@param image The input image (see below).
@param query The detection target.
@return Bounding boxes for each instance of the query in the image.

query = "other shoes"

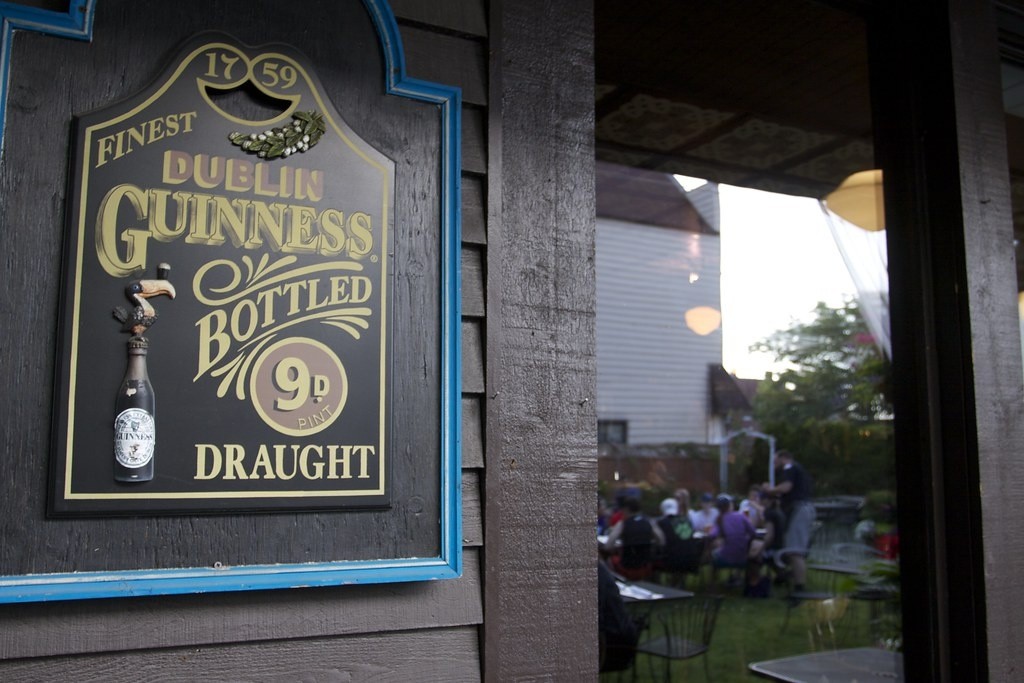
[726,573,737,587]
[788,594,805,610]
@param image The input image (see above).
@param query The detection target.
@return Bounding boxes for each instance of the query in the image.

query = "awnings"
[713,364,751,414]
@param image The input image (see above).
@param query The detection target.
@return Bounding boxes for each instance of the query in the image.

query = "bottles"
[113,336,155,482]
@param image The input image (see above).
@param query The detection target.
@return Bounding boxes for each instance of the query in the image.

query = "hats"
[716,493,734,505]
[701,493,713,502]
[660,498,678,516]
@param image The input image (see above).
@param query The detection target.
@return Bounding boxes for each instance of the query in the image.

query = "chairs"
[611,496,893,683]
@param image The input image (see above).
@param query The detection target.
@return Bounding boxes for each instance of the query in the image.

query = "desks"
[613,579,695,683]
[805,560,895,647]
[814,502,853,548]
[746,647,906,683]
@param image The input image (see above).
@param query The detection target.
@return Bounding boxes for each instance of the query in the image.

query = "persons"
[597,451,816,607]
